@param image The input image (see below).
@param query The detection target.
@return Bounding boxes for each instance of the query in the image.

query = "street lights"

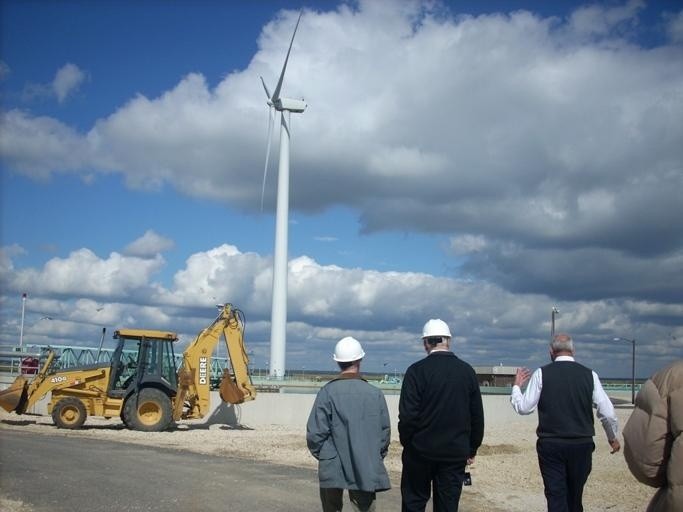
[550,306,560,344]
[18,293,27,353]
[613,337,635,404]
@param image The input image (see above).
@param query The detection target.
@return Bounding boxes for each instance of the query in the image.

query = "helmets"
[333,336,365,363]
[419,319,452,339]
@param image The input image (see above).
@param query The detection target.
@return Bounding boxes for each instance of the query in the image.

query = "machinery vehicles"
[0,304,256,433]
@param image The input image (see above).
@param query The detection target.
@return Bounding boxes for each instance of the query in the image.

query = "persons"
[306,337,391,512]
[398,318,484,512]
[622,360,683,512]
[509,334,620,512]
